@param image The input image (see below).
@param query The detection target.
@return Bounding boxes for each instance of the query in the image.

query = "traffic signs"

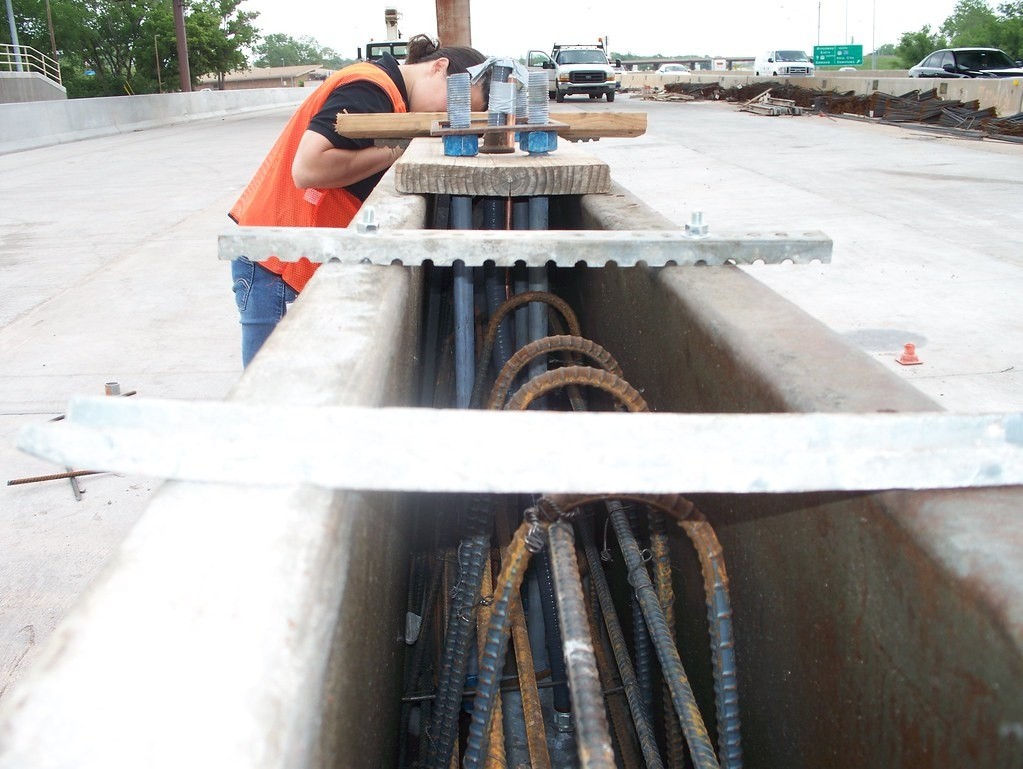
[814,45,864,67]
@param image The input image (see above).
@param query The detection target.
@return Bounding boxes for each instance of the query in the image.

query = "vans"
[753,49,815,78]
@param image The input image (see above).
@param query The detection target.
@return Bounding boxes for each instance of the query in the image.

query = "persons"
[230,32,493,370]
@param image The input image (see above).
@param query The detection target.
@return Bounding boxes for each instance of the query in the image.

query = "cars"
[655,63,692,75]
[908,47,1023,78]
[838,68,857,71]
[609,64,625,72]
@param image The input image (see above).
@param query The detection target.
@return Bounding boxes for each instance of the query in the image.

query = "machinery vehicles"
[354,41,413,65]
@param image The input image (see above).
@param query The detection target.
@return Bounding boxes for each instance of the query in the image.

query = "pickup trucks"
[526,36,616,102]
